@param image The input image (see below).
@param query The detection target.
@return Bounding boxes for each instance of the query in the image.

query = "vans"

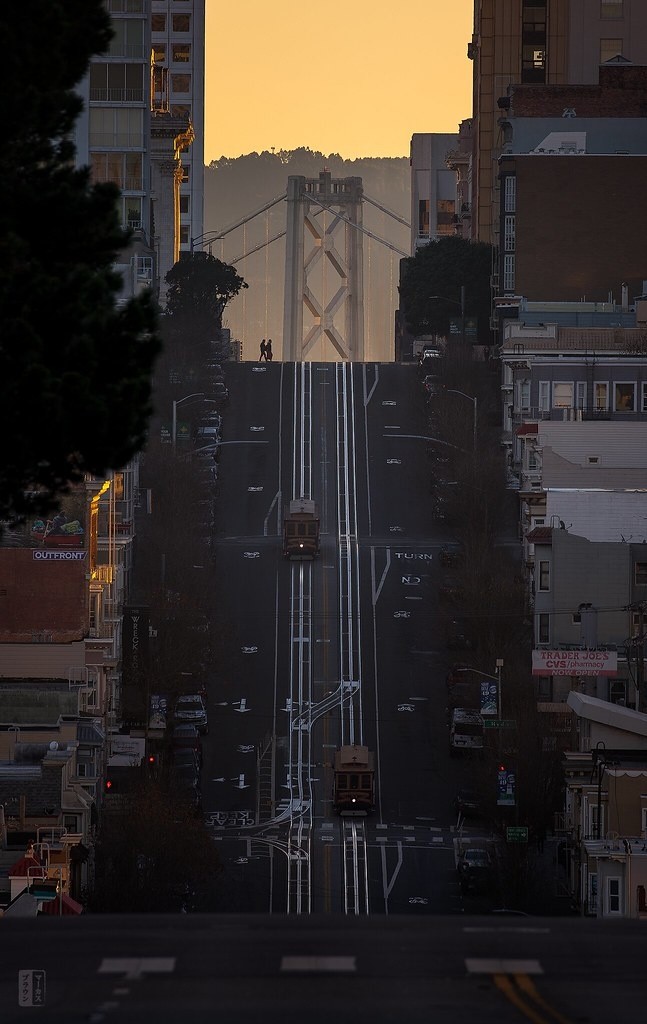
[450,708,485,758]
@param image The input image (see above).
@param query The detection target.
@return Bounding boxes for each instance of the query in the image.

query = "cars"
[456,848,493,881]
[454,790,485,818]
[159,353,230,915]
[417,345,481,709]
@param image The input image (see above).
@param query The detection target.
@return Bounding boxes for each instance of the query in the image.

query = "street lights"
[172,391,270,462]
[447,481,488,535]
[381,388,478,523]
[456,669,502,762]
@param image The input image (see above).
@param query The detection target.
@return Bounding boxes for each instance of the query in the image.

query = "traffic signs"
[507,826,528,843]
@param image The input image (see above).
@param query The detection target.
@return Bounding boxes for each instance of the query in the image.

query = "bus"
[283,499,322,561]
[332,744,376,817]
